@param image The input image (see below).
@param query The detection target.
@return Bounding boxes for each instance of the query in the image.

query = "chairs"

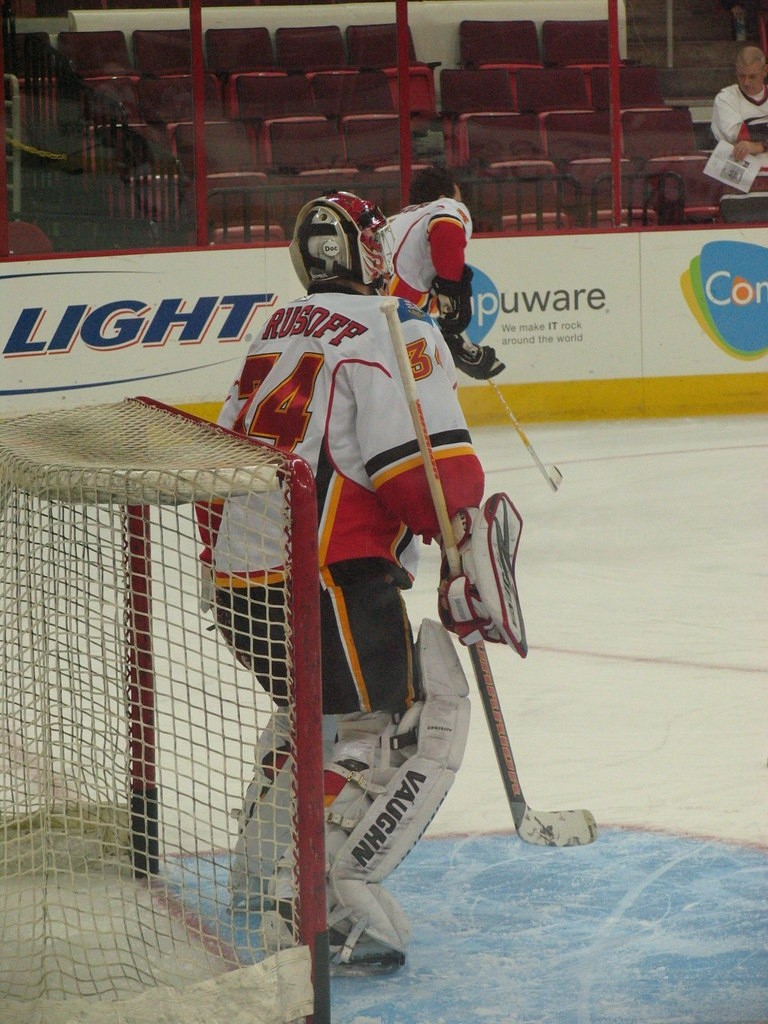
[0,21,767,256]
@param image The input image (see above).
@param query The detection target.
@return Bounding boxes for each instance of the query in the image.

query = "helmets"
[288,191,397,294]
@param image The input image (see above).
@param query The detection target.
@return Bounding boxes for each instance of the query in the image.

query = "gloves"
[432,263,473,335]
[443,332,506,381]
[436,506,506,646]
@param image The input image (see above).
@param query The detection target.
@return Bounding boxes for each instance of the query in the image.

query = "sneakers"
[260,880,405,977]
[226,874,268,916]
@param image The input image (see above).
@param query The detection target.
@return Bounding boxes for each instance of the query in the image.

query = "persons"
[385,170,506,379]
[711,47,768,222]
[721,0,768,43]
[197,193,528,975]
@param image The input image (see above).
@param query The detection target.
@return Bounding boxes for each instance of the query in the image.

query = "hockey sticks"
[379,298,597,847]
[488,378,561,490]
